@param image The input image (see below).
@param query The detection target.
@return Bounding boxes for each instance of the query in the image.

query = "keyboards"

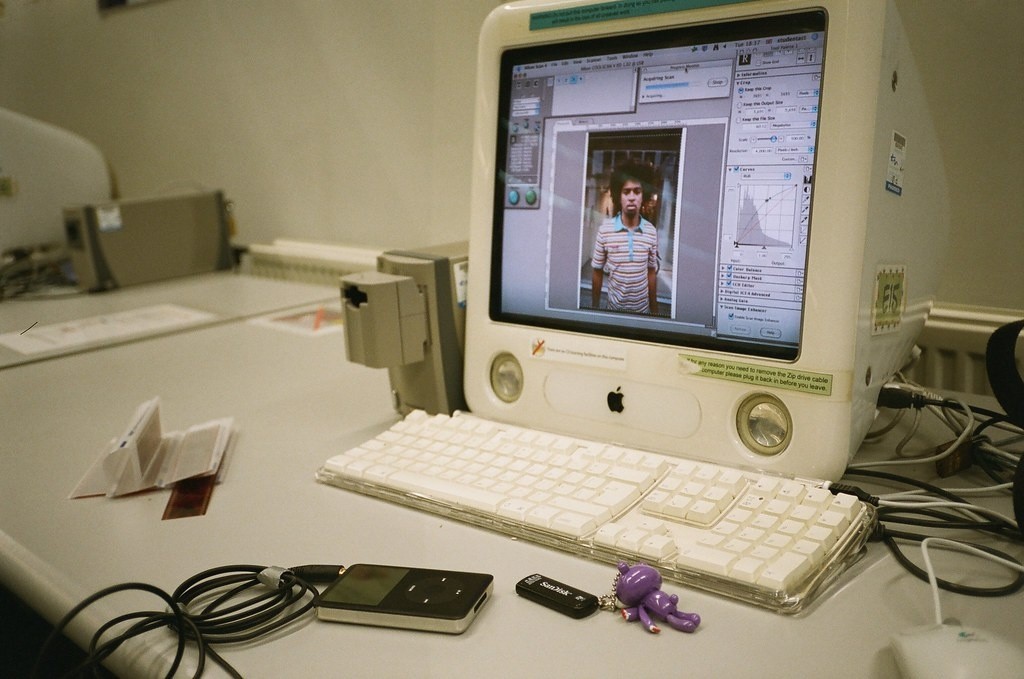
[317,405,873,614]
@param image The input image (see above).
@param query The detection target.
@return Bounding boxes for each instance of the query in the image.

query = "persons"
[591,169,659,315]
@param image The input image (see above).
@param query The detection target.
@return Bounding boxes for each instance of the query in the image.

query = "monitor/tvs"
[0,109,115,281]
[463,0,948,487]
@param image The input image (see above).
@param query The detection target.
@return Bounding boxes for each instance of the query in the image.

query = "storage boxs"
[61,190,237,293]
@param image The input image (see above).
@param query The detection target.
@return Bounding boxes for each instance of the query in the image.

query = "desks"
[0,271,1024,679]
